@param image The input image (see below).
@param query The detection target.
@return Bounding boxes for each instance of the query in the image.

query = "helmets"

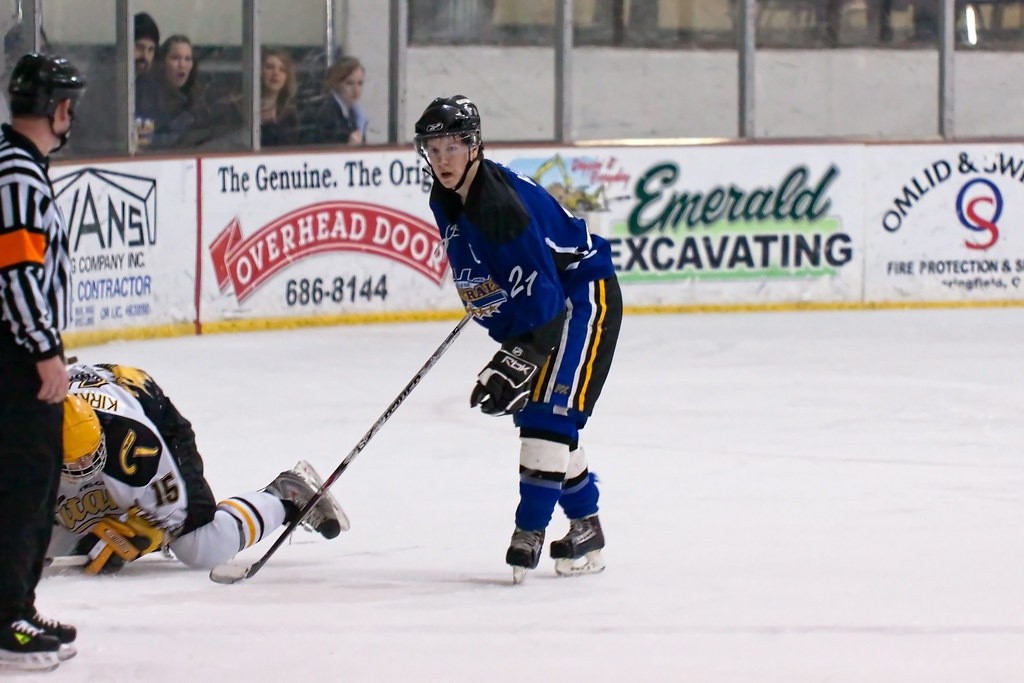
[9,51,86,117]
[413,95,480,159]
[61,394,107,483]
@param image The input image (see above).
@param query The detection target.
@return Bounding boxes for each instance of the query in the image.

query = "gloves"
[75,510,160,576]
[471,333,547,415]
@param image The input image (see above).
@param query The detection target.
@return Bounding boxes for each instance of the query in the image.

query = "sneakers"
[30,613,77,661]
[259,458,349,540]
[549,516,605,576]
[505,525,545,584]
[1,620,61,671]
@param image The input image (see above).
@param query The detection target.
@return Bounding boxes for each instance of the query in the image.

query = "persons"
[0,21,72,158]
[308,57,369,146]
[225,45,302,150]
[70,12,162,158]
[42,364,350,573]
[0,54,88,673]
[413,95,623,586]
[147,33,225,150]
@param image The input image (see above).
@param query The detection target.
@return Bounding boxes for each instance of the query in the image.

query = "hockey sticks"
[43,554,92,568]
[208,308,476,587]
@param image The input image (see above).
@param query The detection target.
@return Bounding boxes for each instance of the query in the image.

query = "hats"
[134,12,161,47]
